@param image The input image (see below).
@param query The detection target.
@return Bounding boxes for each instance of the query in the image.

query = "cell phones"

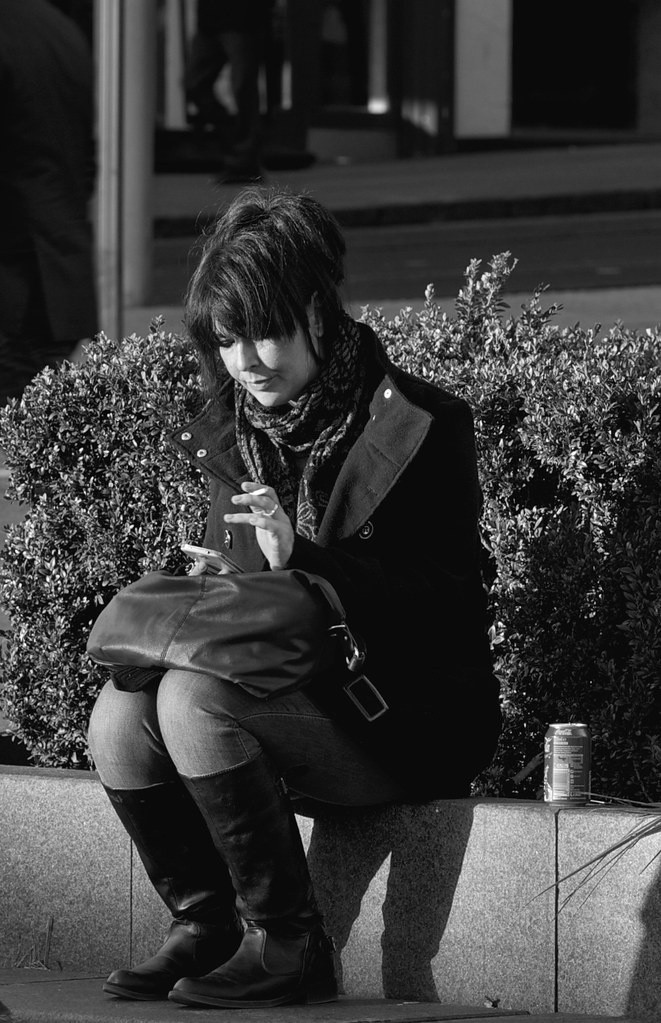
[180,545,243,575]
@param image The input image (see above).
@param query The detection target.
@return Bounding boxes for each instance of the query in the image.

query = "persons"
[89,189,501,1007]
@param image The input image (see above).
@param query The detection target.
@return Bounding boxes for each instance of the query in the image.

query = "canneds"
[543,721,592,808]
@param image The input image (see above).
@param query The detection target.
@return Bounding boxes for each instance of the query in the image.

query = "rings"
[262,503,279,518]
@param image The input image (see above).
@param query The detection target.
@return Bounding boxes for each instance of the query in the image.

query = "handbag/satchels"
[87,572,361,698]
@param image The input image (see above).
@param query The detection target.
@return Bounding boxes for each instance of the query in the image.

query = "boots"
[168,747,336,1007]
[103,778,246,999]
